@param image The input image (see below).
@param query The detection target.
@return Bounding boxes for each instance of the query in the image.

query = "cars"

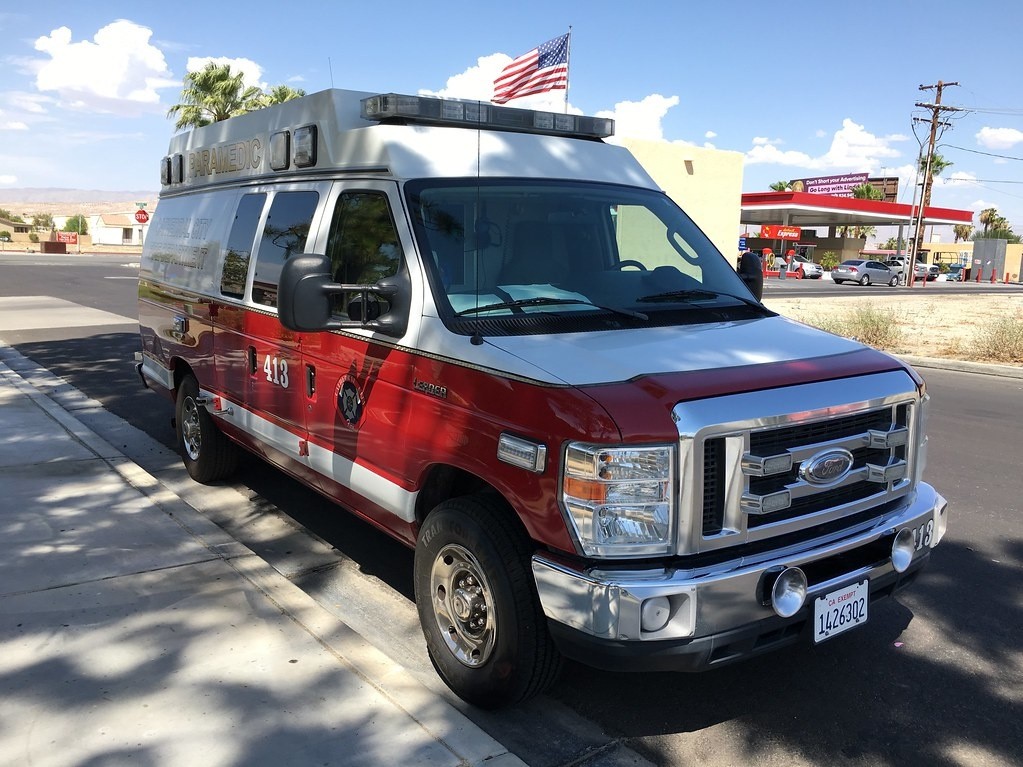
[882,260,919,281]
[831,259,900,287]
[773,254,824,279]
[889,256,940,281]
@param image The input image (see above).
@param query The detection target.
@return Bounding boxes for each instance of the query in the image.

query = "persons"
[354,215,400,273]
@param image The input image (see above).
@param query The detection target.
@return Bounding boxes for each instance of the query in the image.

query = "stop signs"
[135,210,149,223]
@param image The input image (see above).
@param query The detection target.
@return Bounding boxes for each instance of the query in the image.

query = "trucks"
[946,264,967,281]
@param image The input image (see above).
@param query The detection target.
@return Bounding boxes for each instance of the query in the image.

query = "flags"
[490,33,569,104]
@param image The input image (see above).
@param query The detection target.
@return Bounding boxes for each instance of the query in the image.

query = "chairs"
[503,221,603,295]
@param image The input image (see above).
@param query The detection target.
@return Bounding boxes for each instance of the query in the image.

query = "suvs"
[135,87,949,711]
